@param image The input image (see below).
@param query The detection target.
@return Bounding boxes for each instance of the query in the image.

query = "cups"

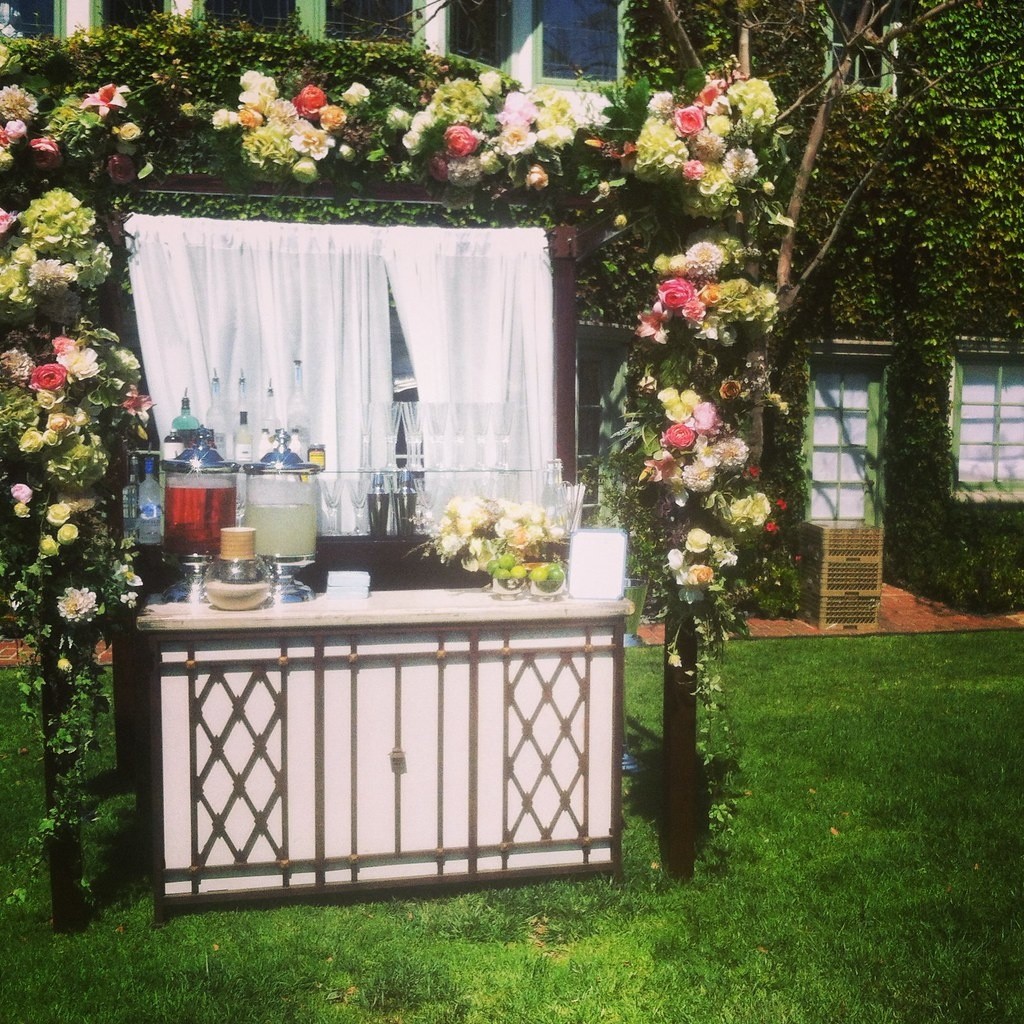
[355,401,513,470]
[320,476,585,537]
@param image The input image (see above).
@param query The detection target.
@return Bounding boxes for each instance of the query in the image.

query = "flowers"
[1,24,800,905]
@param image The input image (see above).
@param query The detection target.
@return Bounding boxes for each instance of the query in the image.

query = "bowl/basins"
[530,580,566,601]
[492,579,525,600]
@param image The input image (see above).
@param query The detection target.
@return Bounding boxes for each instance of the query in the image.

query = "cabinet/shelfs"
[113,591,637,928]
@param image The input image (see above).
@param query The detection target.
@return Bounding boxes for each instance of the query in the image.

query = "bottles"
[233,378,254,431]
[260,388,279,435]
[393,472,417,535]
[287,360,310,443]
[122,456,141,537]
[367,474,390,536]
[205,376,226,456]
[139,458,161,544]
[259,429,273,459]
[204,526,271,610]
[172,397,200,447]
[164,429,185,460]
[542,458,565,531]
[233,412,253,461]
[287,428,302,457]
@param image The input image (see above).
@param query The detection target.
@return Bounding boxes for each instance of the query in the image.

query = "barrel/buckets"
[623,578,648,635]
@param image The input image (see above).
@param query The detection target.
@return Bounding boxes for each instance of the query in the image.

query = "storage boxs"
[799,555,882,598]
[800,521,885,564]
[799,586,881,632]
